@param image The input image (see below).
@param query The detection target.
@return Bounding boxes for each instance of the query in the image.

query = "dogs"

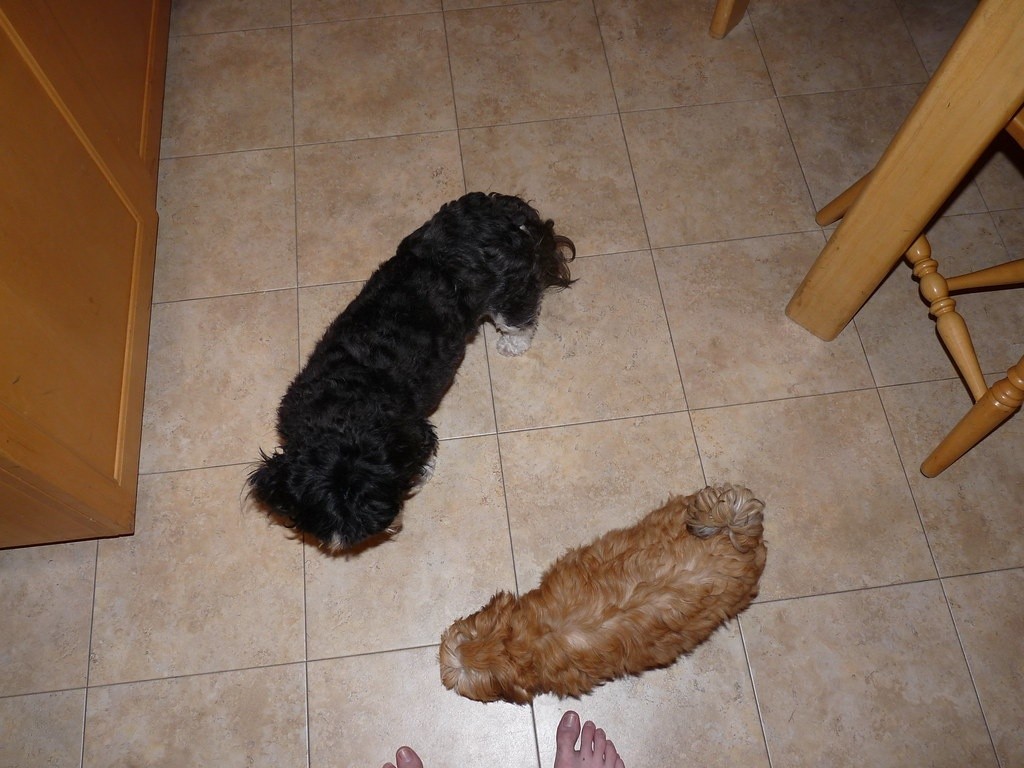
[248,188,578,559]
[439,484,768,706]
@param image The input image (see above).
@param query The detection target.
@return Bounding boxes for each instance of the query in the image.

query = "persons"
[383,710,625,768]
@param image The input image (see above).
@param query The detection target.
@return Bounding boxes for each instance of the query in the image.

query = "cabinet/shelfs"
[0,0,174,551]
